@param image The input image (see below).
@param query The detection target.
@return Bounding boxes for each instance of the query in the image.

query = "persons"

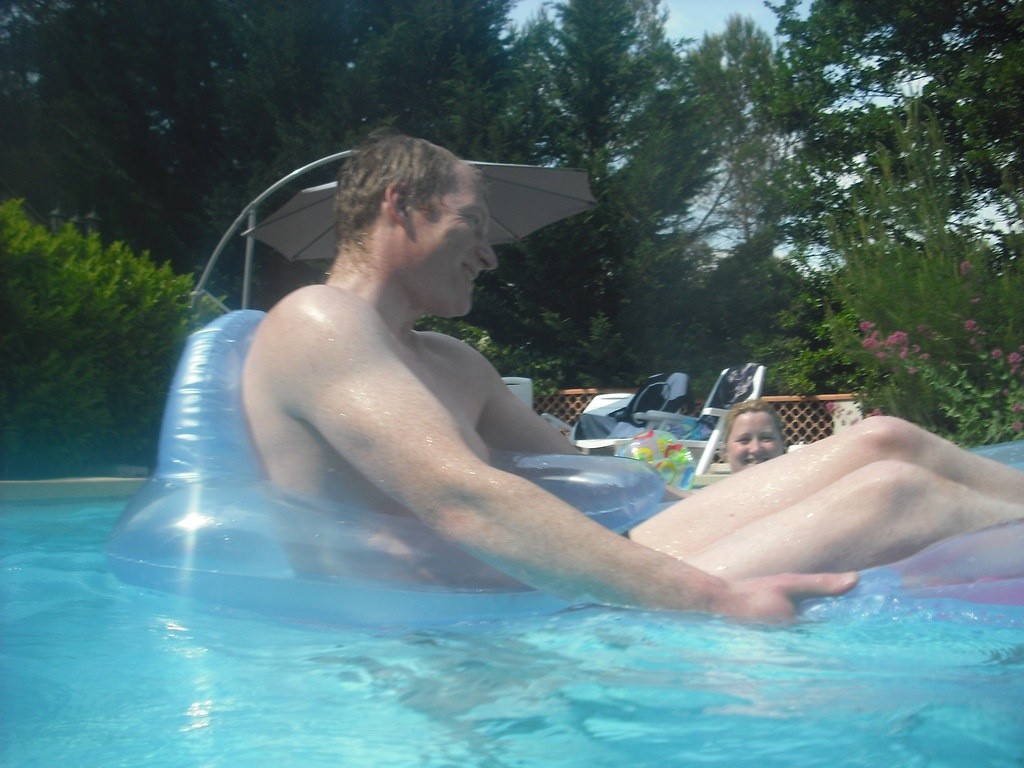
[243,124,1023,631]
[724,401,786,474]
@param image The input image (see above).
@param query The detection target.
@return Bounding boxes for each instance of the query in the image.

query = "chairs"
[569,371,689,457]
[540,393,635,440]
[646,362,771,476]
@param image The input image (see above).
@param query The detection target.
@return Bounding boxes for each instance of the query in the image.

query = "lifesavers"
[107,306,1022,605]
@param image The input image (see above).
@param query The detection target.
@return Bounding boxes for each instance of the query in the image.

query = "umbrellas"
[241,160,599,262]
